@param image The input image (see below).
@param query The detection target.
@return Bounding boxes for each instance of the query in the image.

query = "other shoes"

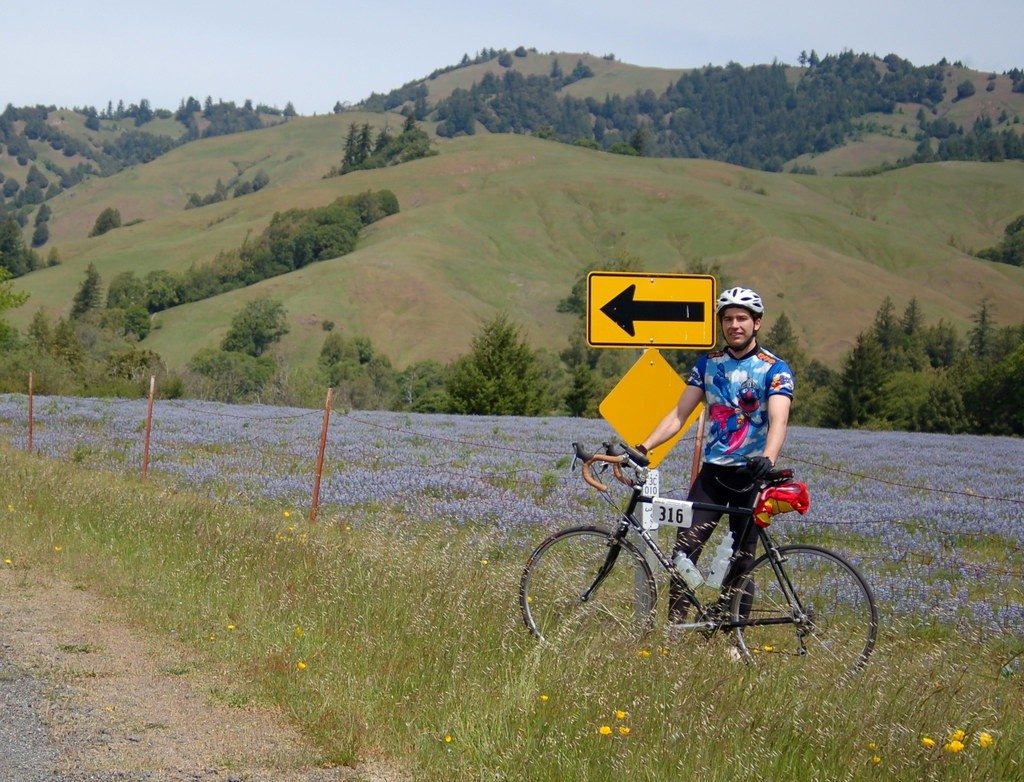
[727,645,742,662]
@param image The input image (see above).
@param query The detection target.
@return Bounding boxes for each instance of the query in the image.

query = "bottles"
[671,549,704,590]
[706,532,734,590]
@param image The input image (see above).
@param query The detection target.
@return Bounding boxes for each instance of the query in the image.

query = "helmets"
[716,287,764,319]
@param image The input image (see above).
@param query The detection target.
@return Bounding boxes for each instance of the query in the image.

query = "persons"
[621,288,793,660]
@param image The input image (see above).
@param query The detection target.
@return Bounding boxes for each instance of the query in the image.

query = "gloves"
[745,456,772,483]
[618,444,650,469]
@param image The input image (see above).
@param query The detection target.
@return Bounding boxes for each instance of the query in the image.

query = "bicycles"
[519,435,880,687]
